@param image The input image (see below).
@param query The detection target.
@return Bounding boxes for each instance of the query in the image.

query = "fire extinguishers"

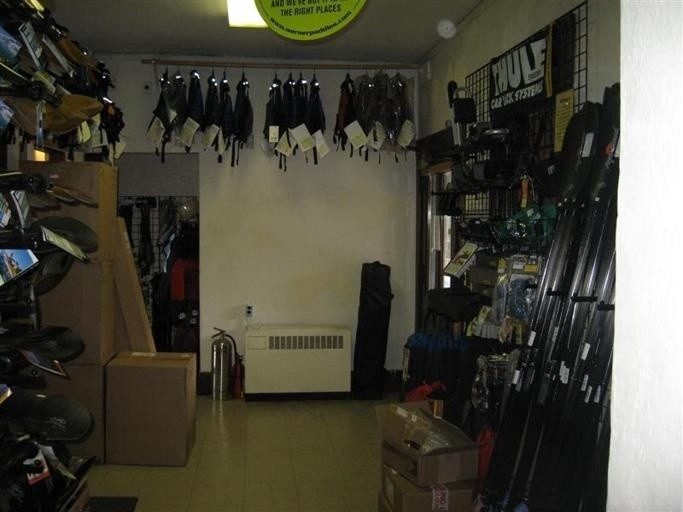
[211,327,243,401]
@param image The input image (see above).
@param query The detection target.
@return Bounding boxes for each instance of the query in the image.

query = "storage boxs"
[104,350,195,467]
[113,219,157,355]
[373,397,488,512]
[29,162,118,468]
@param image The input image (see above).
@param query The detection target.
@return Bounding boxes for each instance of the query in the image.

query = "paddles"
[0,0,103,512]
[474,82,620,512]
